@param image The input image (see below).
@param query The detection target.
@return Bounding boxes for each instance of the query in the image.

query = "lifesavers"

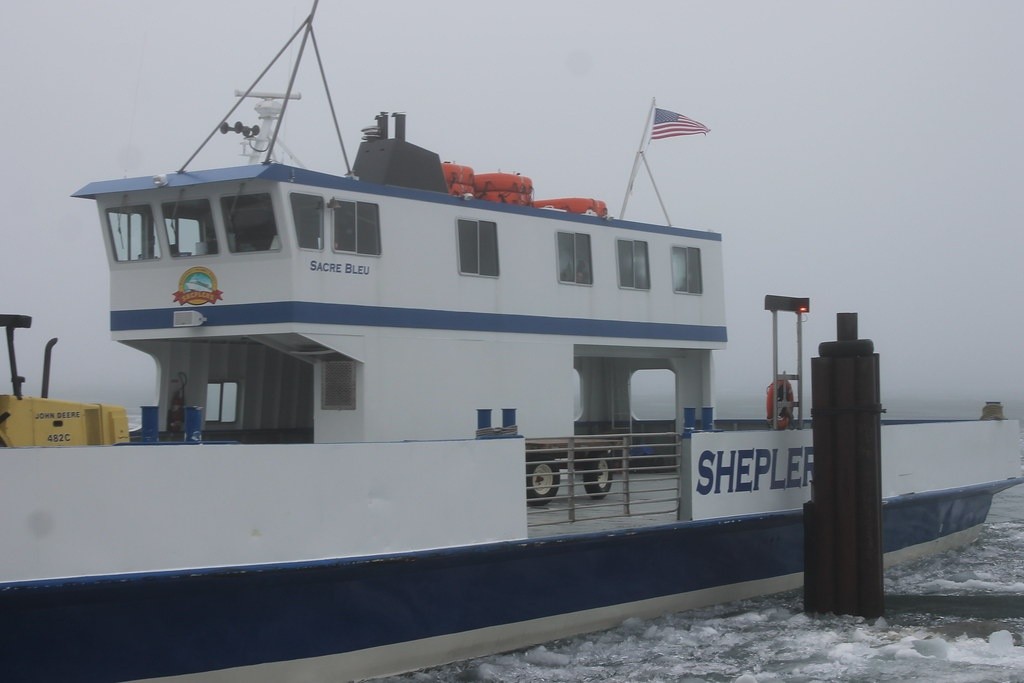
[533,198,608,216]
[445,183,475,197]
[474,172,534,193]
[476,191,531,207]
[442,162,475,185]
[766,380,794,429]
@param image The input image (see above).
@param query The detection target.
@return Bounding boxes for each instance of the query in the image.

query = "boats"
[1,0,1024,683]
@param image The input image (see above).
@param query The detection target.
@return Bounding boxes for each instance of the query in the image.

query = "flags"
[651,107,712,141]
